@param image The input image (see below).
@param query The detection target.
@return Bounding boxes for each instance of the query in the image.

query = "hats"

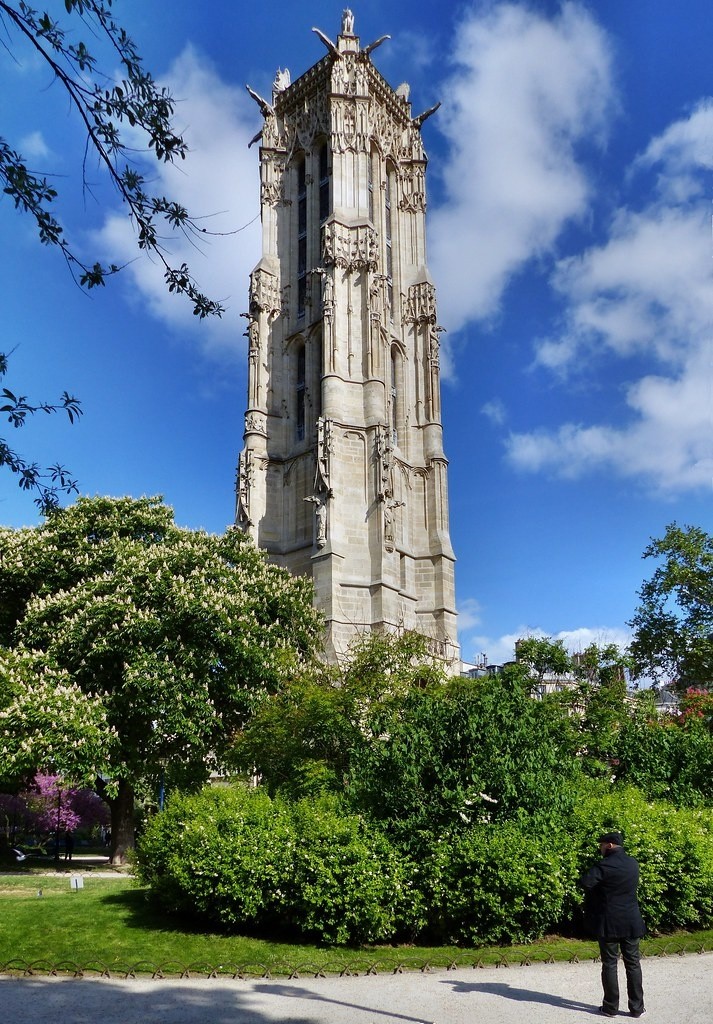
[596,833,623,846]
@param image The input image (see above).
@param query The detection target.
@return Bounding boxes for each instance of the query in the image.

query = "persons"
[64,837,74,860]
[578,831,646,1018]
[102,831,111,847]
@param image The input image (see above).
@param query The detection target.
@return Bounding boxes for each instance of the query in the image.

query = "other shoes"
[637,1008,647,1019]
[599,1005,617,1017]
